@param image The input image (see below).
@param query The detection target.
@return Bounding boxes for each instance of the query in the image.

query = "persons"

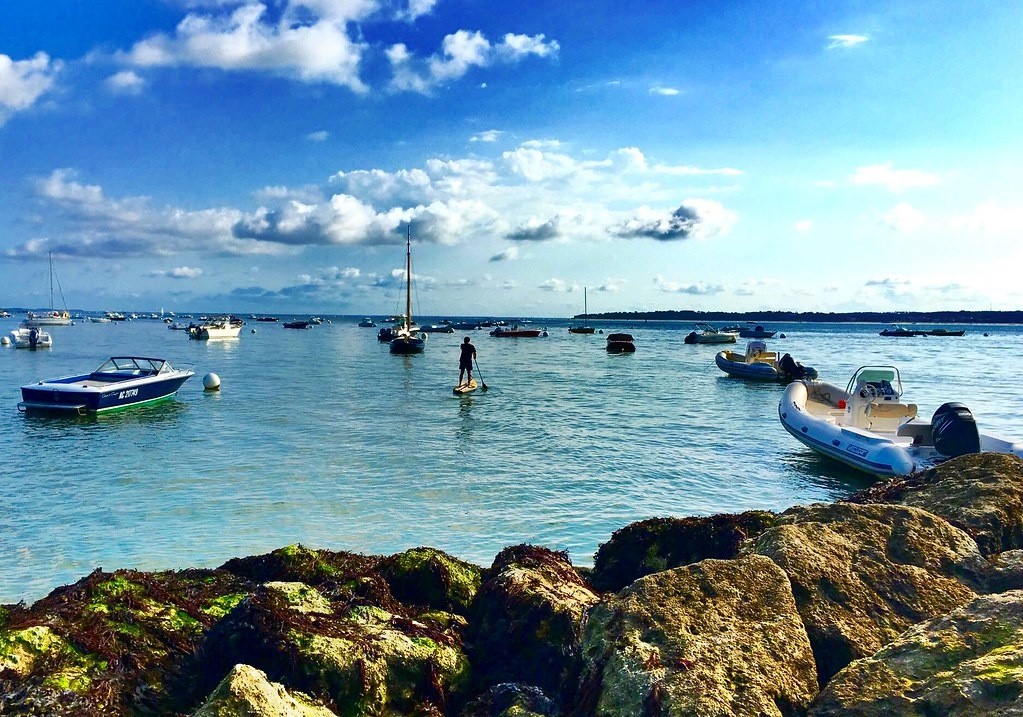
[459,337,476,388]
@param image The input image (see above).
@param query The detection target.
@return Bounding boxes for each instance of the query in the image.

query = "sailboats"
[390,224,426,353]
[568,287,596,334]
[22,250,71,327]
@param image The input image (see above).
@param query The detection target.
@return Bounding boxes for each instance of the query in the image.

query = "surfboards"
[453,378,478,393]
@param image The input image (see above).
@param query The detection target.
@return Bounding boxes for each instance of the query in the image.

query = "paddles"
[474,358,488,389]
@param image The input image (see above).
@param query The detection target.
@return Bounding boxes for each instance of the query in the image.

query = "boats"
[715,340,818,381]
[190,315,244,339]
[606,332,636,353]
[17,354,194,418]
[777,365,1022,480]
[685,323,737,344]
[0,307,542,348]
[721,322,777,338]
[879,324,966,336]
